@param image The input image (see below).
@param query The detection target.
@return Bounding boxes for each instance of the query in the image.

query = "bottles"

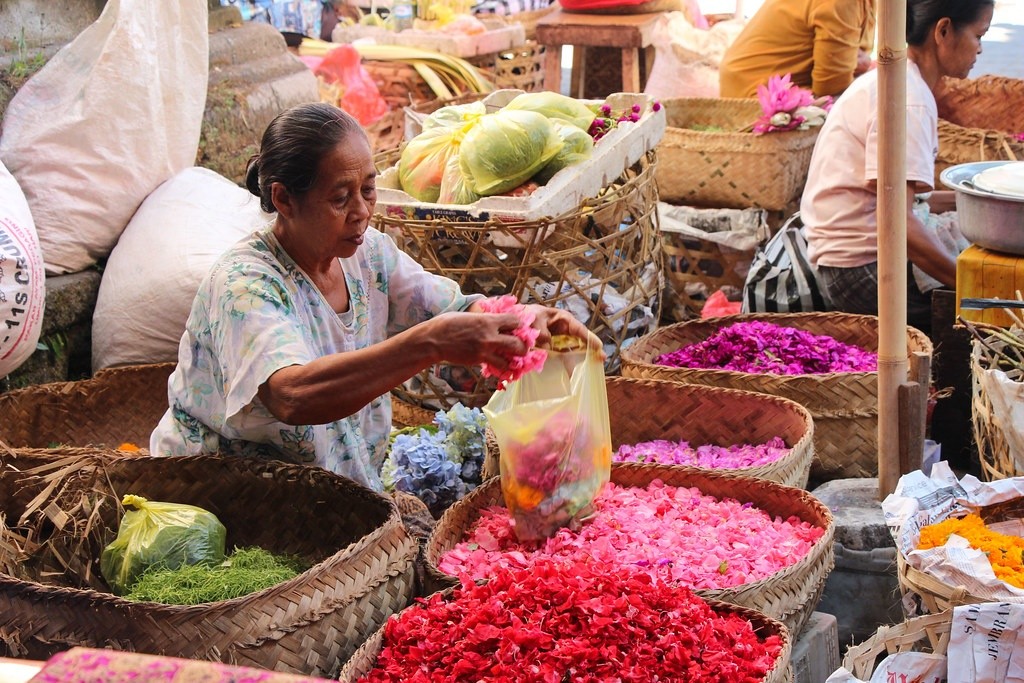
[392,1,412,34]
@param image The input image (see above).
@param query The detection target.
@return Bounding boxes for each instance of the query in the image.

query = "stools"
[535,9,663,95]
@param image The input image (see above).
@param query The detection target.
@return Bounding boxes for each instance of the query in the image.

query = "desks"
[931,244,1024,351]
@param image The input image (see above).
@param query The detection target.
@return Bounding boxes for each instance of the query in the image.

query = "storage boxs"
[790,611,840,683]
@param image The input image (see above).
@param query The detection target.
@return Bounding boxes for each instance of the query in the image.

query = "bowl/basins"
[939,161,1024,258]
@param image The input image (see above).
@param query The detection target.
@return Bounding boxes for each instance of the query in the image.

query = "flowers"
[590,97,661,141]
[742,73,834,136]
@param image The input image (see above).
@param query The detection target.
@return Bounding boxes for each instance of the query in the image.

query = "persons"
[718,0,876,99]
[800,0,995,326]
[147,100,608,494]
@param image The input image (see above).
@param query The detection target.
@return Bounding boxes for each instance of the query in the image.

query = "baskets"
[422,458,837,648]
[831,603,1024,683]
[336,578,792,683]
[932,73,1024,167]
[0,360,180,512]
[895,472,1024,624]
[658,202,802,323]
[364,146,668,431]
[603,373,816,491]
[309,0,567,160]
[950,287,1024,483]
[0,453,420,681]
[651,96,829,212]
[623,309,935,482]
